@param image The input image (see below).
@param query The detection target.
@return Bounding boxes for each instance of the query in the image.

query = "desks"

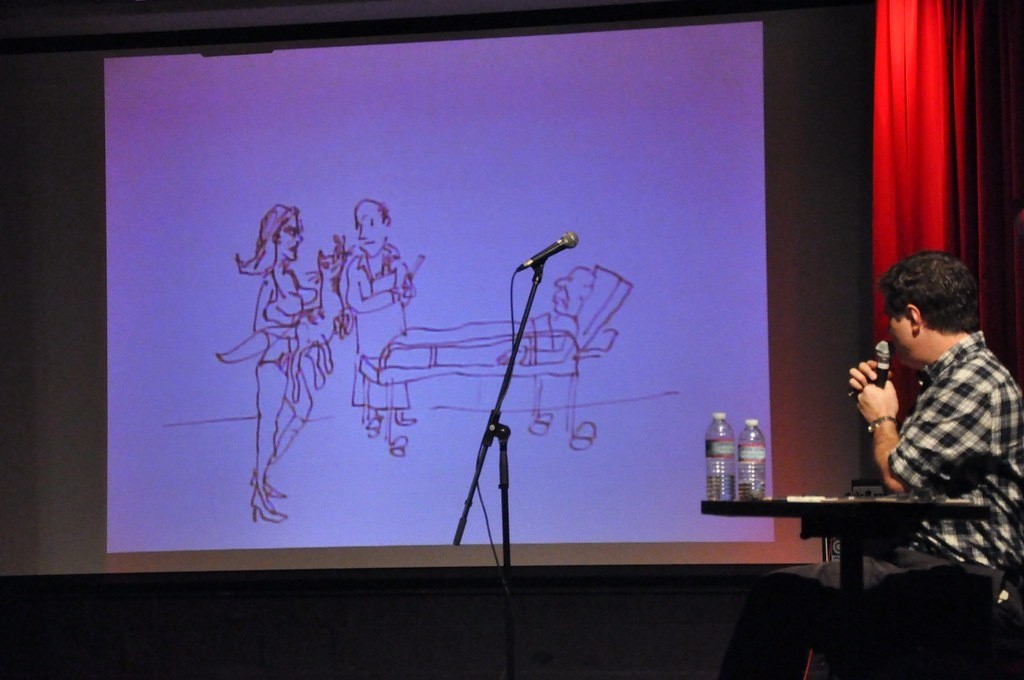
[702,498,992,680]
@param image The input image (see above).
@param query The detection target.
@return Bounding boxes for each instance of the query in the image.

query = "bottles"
[705,413,737,503]
[737,419,766,501]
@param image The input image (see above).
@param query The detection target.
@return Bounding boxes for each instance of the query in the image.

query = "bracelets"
[869,416,897,432]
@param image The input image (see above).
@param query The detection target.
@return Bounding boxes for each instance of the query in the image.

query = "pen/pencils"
[786,496,855,502]
[847,366,895,397]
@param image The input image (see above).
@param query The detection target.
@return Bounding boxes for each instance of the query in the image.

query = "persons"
[722,250,1024,679]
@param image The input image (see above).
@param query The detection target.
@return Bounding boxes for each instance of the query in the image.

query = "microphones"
[516,231,578,273]
[874,340,894,389]
